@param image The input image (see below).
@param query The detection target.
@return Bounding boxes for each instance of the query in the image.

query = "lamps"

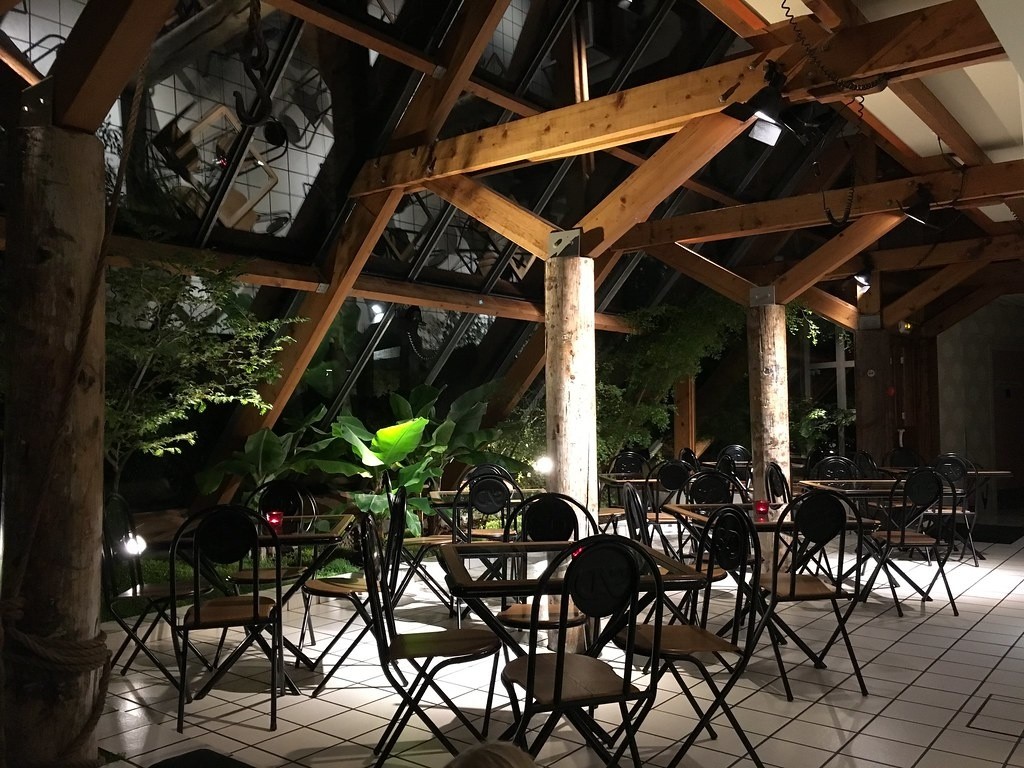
[746,60,803,147]
[854,266,874,286]
[904,183,931,226]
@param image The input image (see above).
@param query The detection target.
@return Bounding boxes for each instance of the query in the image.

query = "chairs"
[103,444,990,767]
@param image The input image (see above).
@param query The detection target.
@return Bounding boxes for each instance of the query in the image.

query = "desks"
[880,466,1012,552]
[798,479,966,580]
[700,460,801,470]
[661,501,880,646]
[597,472,665,543]
[428,488,548,619]
[153,515,355,700]
[438,540,709,750]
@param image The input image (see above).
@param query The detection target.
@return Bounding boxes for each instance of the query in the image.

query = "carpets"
[925,523,1024,544]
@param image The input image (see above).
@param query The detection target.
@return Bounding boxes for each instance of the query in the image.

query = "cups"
[569,541,586,559]
[754,500,770,515]
[266,511,284,528]
